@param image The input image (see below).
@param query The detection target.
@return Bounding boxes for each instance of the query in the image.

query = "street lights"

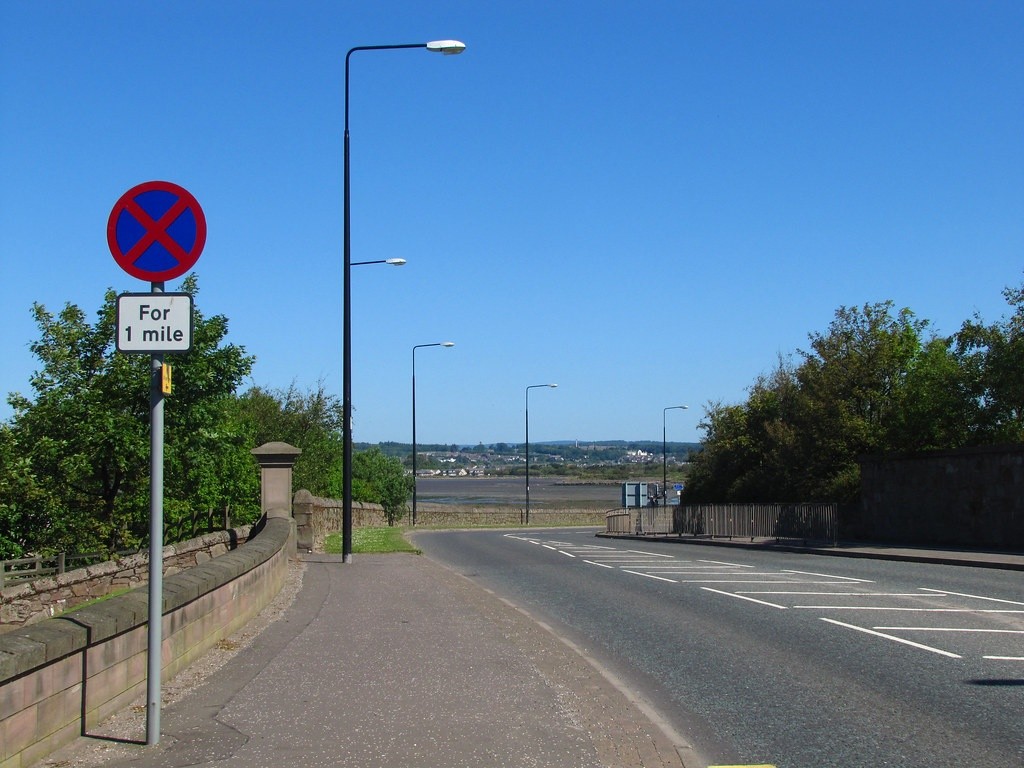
[342,38,467,564]
[339,258,409,563]
[663,405,688,506]
[525,383,559,525]
[412,342,456,528]
